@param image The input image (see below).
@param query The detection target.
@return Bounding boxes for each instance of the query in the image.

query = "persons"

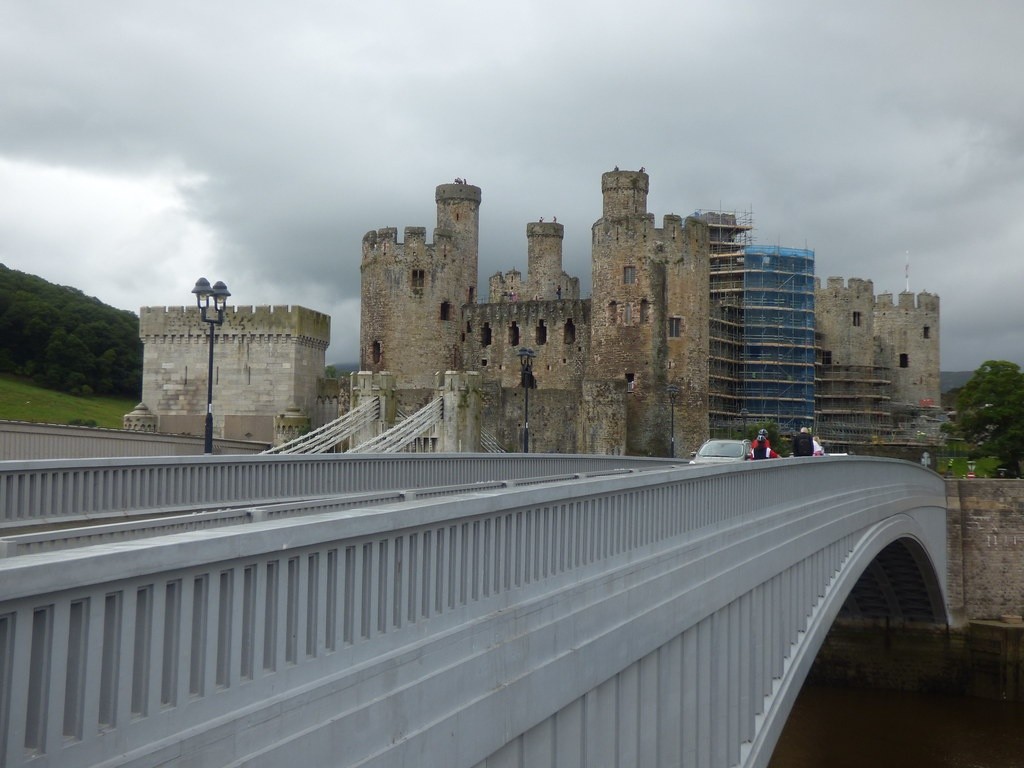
[556,284,561,299]
[502,286,515,302]
[750,428,782,460]
[552,216,557,224]
[792,427,824,457]
[943,458,954,479]
[539,216,544,223]
[614,165,618,171]
[639,167,645,173]
[455,177,466,185]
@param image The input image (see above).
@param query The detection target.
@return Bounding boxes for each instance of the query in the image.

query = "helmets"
[759,429,768,438]
[757,434,766,442]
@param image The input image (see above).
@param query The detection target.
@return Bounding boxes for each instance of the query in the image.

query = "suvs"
[689,436,753,467]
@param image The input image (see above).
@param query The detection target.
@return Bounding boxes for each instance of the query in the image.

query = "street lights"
[516,347,537,453]
[666,384,680,458]
[191,278,232,454]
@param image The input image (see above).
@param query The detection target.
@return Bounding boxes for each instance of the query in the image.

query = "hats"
[801,427,808,433]
